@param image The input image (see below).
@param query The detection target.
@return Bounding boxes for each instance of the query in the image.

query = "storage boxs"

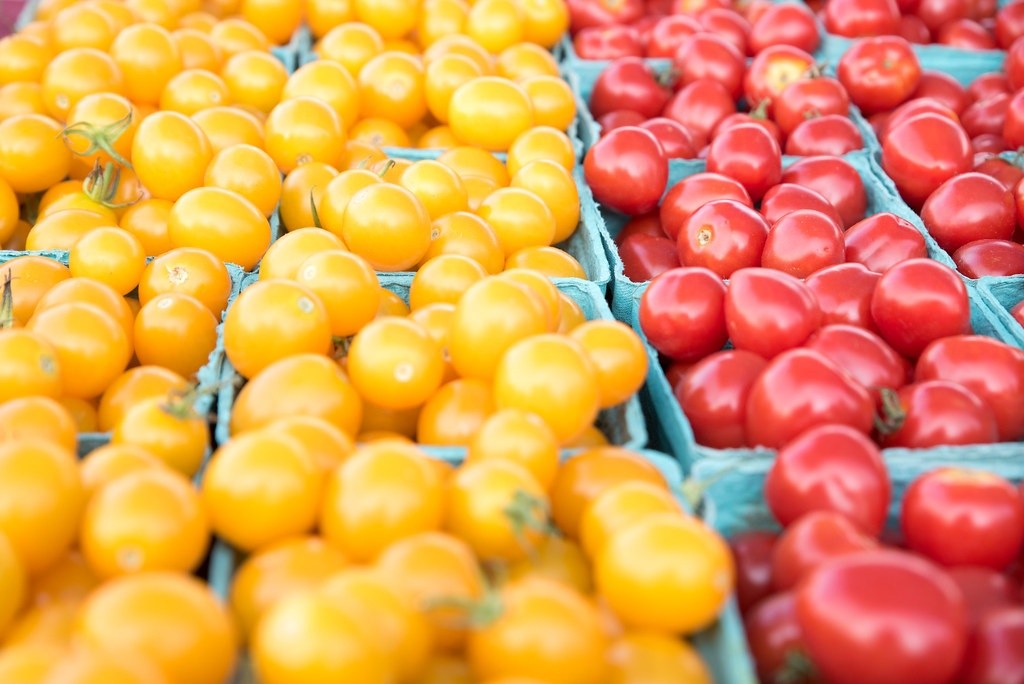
[0,0,1024,684]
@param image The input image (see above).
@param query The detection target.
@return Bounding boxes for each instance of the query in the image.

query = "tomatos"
[565,0,1024,684]
[0,0,736,684]
